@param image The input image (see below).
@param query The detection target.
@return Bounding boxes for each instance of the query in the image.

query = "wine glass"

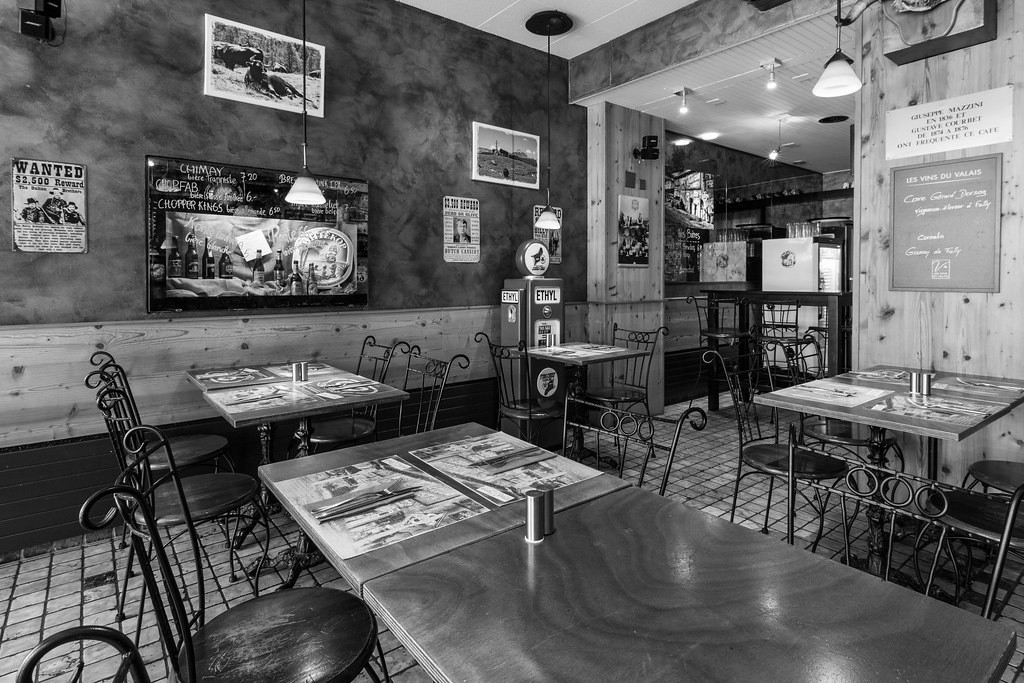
[716,228,748,260]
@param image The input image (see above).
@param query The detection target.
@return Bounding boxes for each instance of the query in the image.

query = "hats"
[49,188,63,195]
[24,198,39,204]
[65,202,78,209]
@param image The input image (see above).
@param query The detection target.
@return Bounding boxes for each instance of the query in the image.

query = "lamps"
[525,10,574,230]
[680,91,688,113]
[285,0,327,205]
[769,119,781,160]
[768,64,776,89]
[811,0,862,98]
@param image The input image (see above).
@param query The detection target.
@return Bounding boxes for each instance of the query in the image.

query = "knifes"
[335,384,379,391]
[226,396,283,406]
[315,486,423,518]
[467,447,539,466]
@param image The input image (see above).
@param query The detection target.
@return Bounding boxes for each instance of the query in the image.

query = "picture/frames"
[204,12,326,119]
[471,120,540,191]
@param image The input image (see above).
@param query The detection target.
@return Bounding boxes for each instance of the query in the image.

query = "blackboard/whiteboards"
[144,154,370,315]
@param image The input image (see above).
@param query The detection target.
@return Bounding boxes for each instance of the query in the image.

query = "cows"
[214,40,264,70]
[245,55,304,100]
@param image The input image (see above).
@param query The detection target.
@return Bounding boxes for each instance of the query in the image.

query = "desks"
[186,360,349,552]
[754,363,1024,541]
[202,372,411,592]
[512,341,652,471]
[258,423,635,600]
[363,485,1018,683]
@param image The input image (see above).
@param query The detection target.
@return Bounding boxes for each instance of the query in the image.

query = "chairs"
[16,294,1024,683]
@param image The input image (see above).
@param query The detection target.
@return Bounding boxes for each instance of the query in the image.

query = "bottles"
[202,237,215,279]
[536,485,556,536]
[910,372,931,395]
[273,249,285,287]
[252,250,265,287]
[168,236,182,278]
[307,263,318,295]
[219,247,234,279]
[300,361,308,381]
[524,490,545,543]
[185,234,199,279]
[292,363,299,382]
[291,260,302,296]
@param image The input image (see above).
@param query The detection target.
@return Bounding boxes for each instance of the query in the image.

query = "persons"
[453,218,471,243]
[64,202,85,226]
[20,198,44,223]
[41,188,68,224]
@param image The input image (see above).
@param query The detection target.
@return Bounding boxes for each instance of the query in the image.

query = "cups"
[786,222,821,238]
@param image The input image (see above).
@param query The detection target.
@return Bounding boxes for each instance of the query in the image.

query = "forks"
[906,398,965,416]
[481,451,545,468]
[238,389,280,401]
[311,476,404,515]
[334,381,371,387]
[956,377,1024,393]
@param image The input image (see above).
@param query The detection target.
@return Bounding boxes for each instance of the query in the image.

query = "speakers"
[640,135,659,159]
[18,0,61,42]
